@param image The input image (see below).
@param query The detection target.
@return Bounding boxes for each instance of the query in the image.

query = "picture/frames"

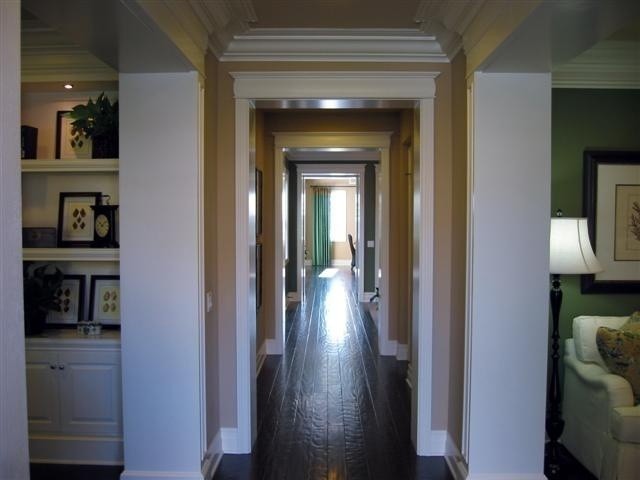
[89,275,121,331]
[57,192,102,247]
[580,150,640,294]
[42,274,85,329]
[56,110,93,159]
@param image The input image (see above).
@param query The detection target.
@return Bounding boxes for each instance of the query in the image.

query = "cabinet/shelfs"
[20,68,124,466]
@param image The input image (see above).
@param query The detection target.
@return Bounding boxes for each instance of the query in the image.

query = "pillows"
[596,311,640,406]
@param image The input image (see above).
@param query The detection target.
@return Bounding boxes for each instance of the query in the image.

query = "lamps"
[549,209,604,480]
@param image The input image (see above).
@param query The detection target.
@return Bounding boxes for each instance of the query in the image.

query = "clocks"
[89,205,120,248]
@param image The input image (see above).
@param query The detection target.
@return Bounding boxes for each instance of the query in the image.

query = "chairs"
[348,234,355,270]
[563,315,640,480]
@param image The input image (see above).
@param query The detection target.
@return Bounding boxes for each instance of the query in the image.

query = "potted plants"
[22,261,65,336]
[70,91,119,159]
